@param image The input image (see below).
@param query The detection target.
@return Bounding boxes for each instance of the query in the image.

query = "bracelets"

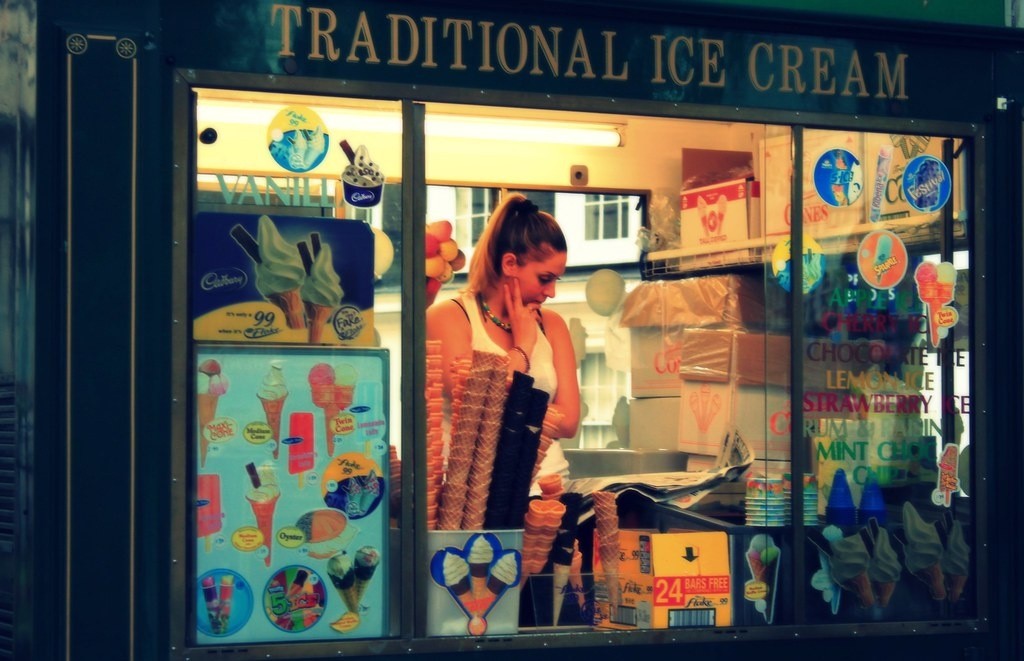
[512,344,530,375]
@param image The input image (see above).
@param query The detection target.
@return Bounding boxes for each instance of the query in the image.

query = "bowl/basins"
[744,469,821,527]
[343,166,385,208]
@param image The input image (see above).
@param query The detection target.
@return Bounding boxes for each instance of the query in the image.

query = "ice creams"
[196,118,520,636]
[744,259,971,607]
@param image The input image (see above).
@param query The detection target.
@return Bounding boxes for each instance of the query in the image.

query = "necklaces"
[475,290,513,331]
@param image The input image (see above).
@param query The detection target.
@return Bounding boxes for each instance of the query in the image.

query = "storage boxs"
[614,124,966,484]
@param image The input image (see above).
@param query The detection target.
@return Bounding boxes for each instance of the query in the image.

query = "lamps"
[194,96,624,153]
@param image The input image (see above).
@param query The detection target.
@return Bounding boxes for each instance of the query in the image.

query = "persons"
[426,189,581,499]
